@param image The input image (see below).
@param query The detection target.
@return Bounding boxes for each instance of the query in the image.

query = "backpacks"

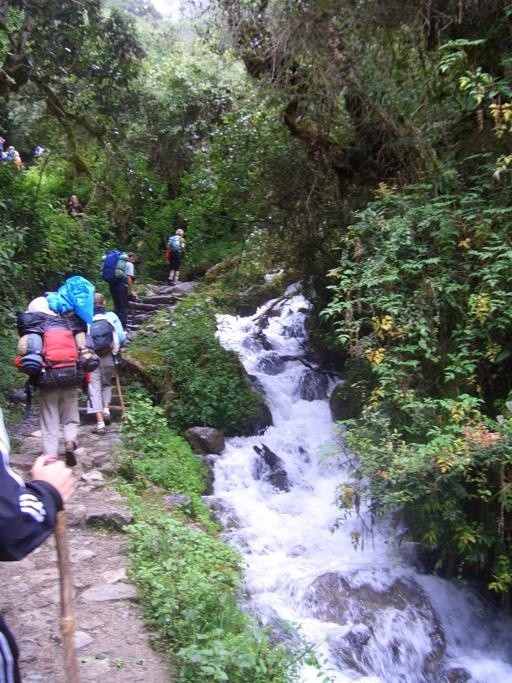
[33,315,84,389]
[102,251,120,283]
[90,320,114,351]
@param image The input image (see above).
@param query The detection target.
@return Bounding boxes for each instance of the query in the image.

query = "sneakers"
[66,446,76,465]
[104,413,110,425]
[95,425,105,434]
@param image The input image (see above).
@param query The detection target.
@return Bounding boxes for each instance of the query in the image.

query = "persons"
[103,246,138,328]
[1,405,78,682]
[18,296,81,467]
[167,228,186,284]
[83,292,128,436]
